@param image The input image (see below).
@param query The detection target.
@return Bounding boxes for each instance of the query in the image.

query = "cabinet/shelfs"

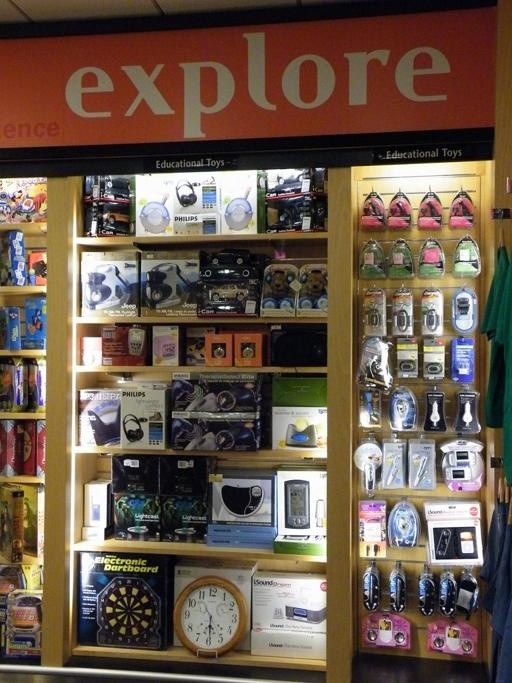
[0,159,512,683]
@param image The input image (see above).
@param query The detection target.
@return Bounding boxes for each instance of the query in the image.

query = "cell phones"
[285,480,310,529]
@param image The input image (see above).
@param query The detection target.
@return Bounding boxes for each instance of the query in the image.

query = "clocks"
[172,576,247,657]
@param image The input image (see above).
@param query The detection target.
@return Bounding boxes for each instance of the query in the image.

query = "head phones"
[176,179,197,207]
[123,414,143,442]
